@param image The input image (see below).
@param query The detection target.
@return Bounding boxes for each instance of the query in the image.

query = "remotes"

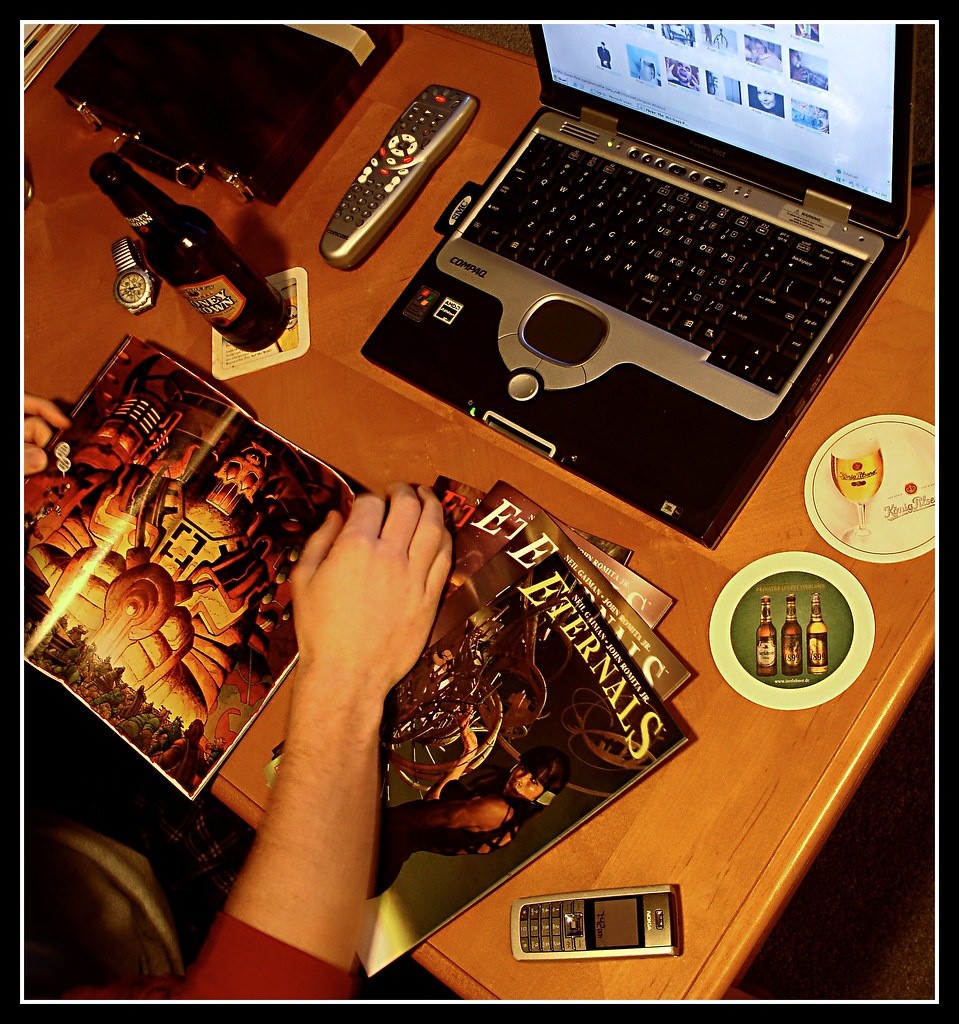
[319,84,477,270]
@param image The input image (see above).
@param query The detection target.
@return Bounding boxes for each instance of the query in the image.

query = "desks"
[25,24,933,1000]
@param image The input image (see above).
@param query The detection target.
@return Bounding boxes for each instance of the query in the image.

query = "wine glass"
[831,447,883,544]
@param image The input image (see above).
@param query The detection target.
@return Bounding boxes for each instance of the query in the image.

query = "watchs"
[111,237,158,315]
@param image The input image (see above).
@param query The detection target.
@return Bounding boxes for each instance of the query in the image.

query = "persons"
[595,24,829,133]
[25,394,453,1000]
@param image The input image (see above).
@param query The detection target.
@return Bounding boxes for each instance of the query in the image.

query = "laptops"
[359,23,923,549]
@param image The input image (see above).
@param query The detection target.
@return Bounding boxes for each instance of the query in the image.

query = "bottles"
[780,597,803,676]
[90,155,290,352]
[756,598,777,676]
[806,593,828,674]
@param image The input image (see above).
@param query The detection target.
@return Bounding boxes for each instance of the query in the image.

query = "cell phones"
[510,885,680,961]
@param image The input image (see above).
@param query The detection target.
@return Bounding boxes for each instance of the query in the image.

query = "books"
[26,334,692,977]
[24,25,80,91]
